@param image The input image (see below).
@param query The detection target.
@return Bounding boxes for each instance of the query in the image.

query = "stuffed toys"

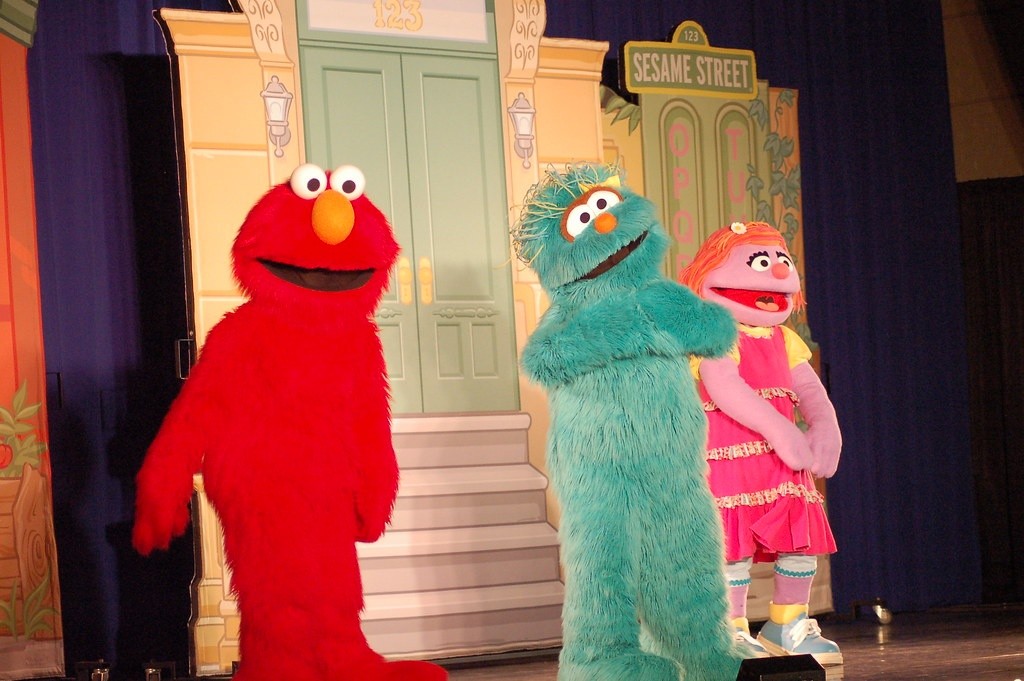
[672,216,847,666]
[130,162,455,679]
[511,157,765,680]
